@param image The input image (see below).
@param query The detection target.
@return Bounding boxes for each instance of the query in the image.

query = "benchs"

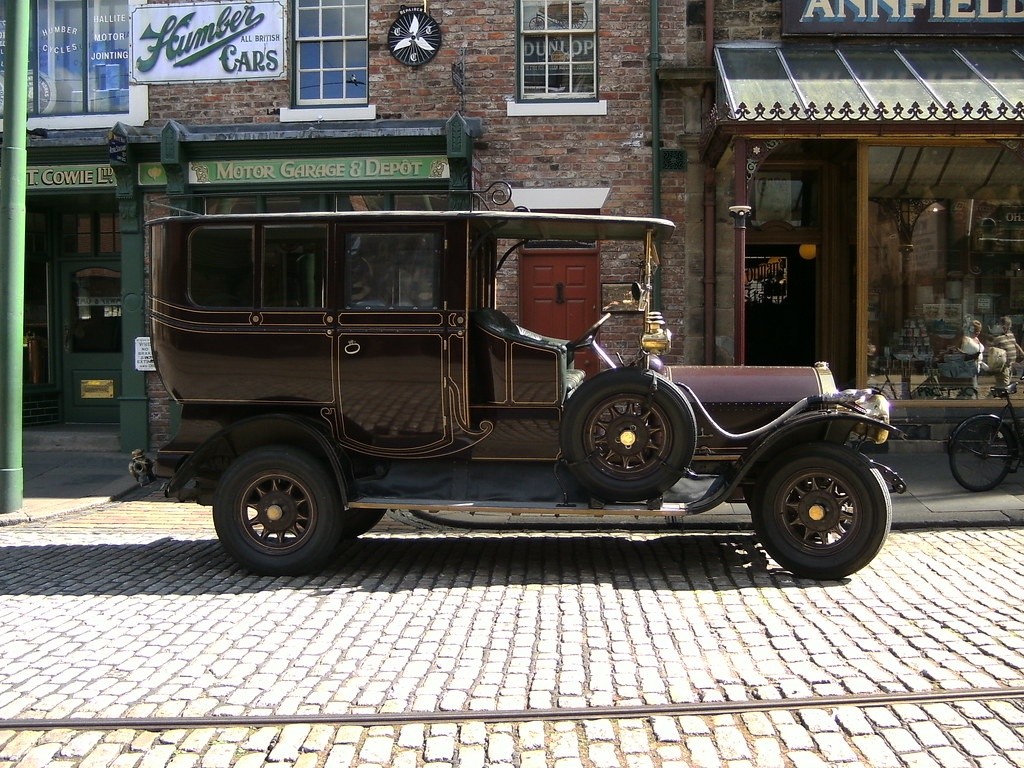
[475,307,586,407]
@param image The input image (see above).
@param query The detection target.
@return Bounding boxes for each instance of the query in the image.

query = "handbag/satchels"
[1016,343,1024,363]
[966,351,980,360]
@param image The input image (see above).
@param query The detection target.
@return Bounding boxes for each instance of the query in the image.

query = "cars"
[139,207,912,582]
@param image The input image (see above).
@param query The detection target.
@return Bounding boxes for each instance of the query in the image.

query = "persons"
[960,315,1024,399]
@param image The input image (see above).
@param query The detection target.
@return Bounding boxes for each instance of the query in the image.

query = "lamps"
[799,244,816,259]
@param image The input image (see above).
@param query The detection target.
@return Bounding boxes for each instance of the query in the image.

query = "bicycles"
[867,365,899,401]
[945,375,1024,492]
